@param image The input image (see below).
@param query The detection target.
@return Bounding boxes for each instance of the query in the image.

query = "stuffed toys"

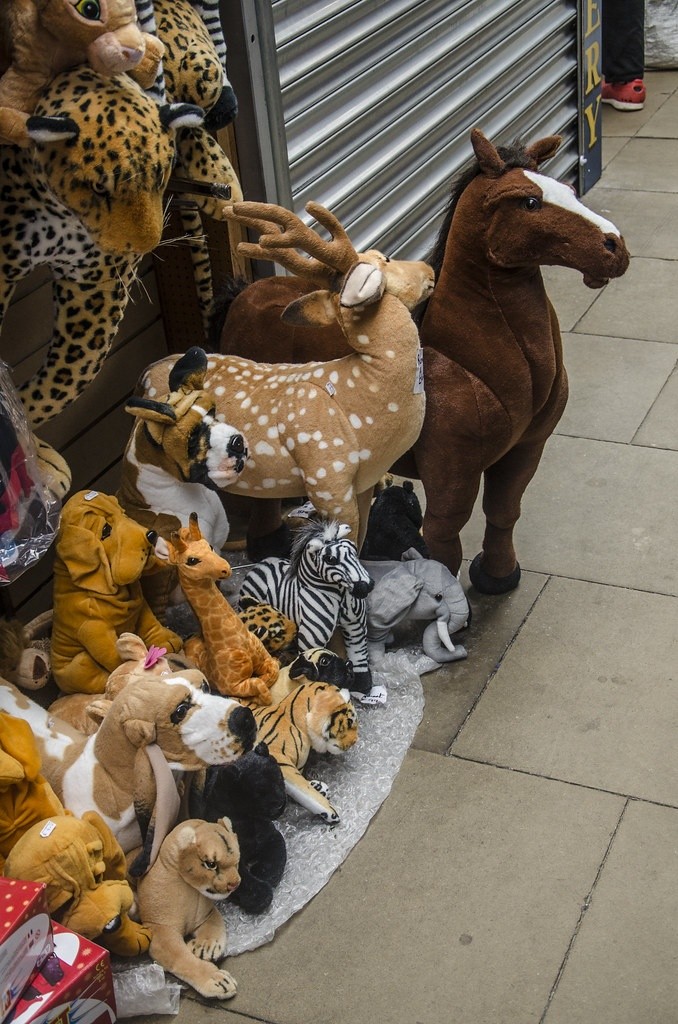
[0,0,628,1001]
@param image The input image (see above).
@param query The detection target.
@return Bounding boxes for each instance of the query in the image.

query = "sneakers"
[601,79,646,110]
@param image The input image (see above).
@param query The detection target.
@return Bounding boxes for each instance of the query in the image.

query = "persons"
[601,0,646,112]
